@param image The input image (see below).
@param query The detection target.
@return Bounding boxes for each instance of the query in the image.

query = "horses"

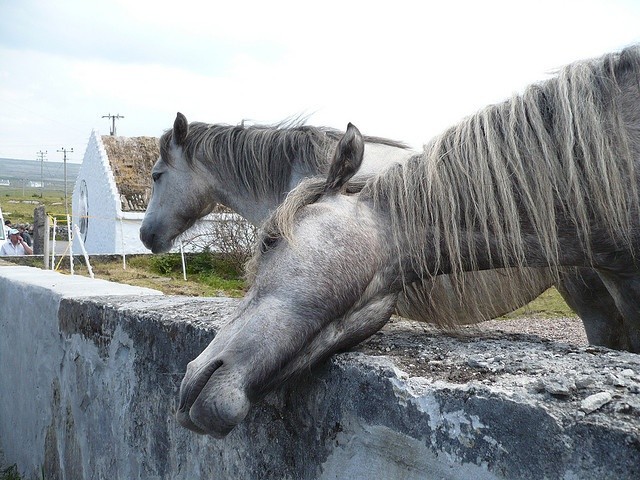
[139,110,627,354]
[176,45,640,439]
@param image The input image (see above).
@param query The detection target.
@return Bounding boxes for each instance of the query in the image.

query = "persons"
[20,227,31,247]
[0,229,33,256]
[5,220,12,239]
